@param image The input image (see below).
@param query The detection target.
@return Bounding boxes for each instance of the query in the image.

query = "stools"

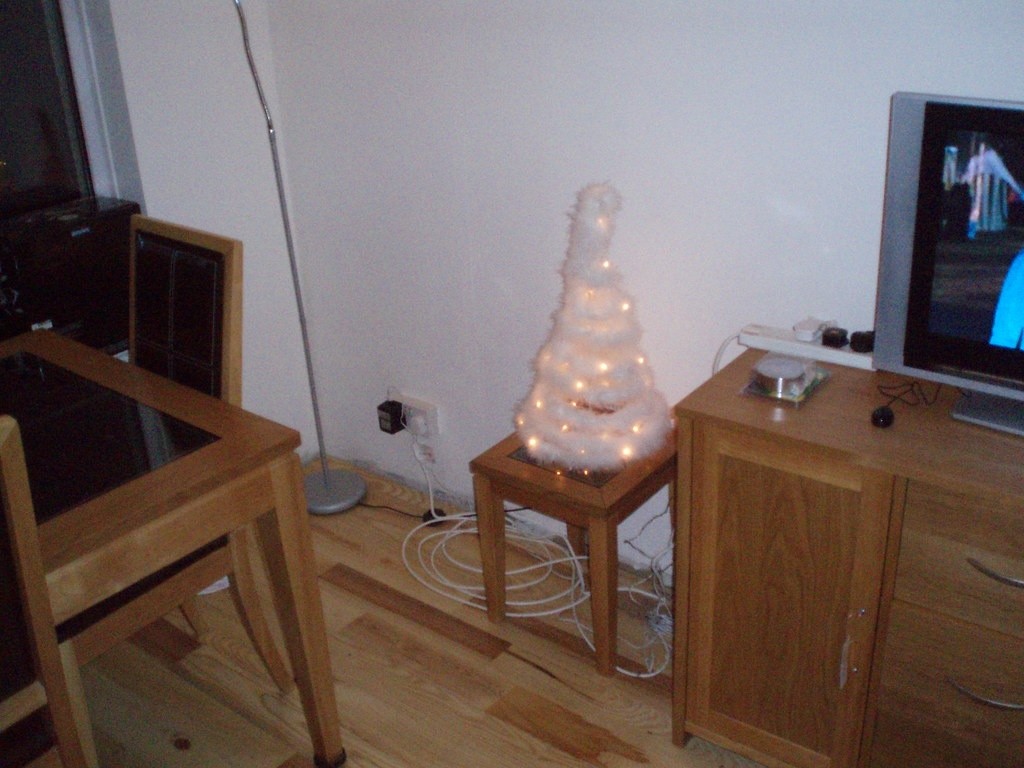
[468,430,677,679]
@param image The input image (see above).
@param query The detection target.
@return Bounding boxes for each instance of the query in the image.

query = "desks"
[0,328,347,768]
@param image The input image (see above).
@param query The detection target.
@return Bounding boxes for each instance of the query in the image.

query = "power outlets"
[391,392,440,439]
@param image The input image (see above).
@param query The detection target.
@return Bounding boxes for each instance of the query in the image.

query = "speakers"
[0,194,142,358]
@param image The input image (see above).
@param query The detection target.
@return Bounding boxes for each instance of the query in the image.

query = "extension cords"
[739,323,879,372]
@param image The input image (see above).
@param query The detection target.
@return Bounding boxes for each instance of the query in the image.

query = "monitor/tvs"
[872,92,1024,437]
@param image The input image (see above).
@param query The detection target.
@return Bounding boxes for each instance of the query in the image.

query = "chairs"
[56,214,294,695]
[0,413,88,768]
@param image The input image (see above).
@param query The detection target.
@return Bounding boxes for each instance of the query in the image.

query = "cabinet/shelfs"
[673,348,1024,768]
